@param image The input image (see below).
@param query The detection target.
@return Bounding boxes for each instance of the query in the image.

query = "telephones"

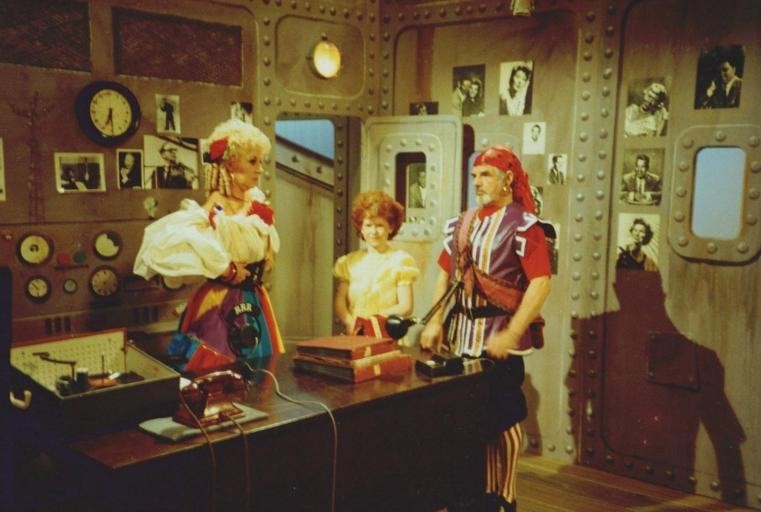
[172,371,247,428]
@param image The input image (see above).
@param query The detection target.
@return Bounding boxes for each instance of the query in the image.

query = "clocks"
[79,79,142,146]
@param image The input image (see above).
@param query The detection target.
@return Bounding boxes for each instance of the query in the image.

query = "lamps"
[308,31,344,81]
[382,268,466,374]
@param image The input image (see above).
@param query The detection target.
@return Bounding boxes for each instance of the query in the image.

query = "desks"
[0,367,502,510]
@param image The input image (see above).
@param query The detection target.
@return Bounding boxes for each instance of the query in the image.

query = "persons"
[416,103,427,115]
[133,118,282,374]
[498,64,530,115]
[700,61,742,108]
[619,154,663,206]
[623,83,669,138]
[617,217,653,271]
[548,155,566,184]
[150,141,195,188]
[120,153,137,186]
[418,144,552,512]
[450,77,471,116]
[465,77,484,115]
[409,168,425,206]
[524,124,543,153]
[332,189,416,337]
[158,96,175,130]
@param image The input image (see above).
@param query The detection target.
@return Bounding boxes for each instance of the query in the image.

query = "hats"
[642,82,668,103]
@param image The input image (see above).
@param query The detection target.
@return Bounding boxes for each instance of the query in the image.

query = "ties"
[638,179,644,199]
[557,171,560,183]
[724,85,728,103]
